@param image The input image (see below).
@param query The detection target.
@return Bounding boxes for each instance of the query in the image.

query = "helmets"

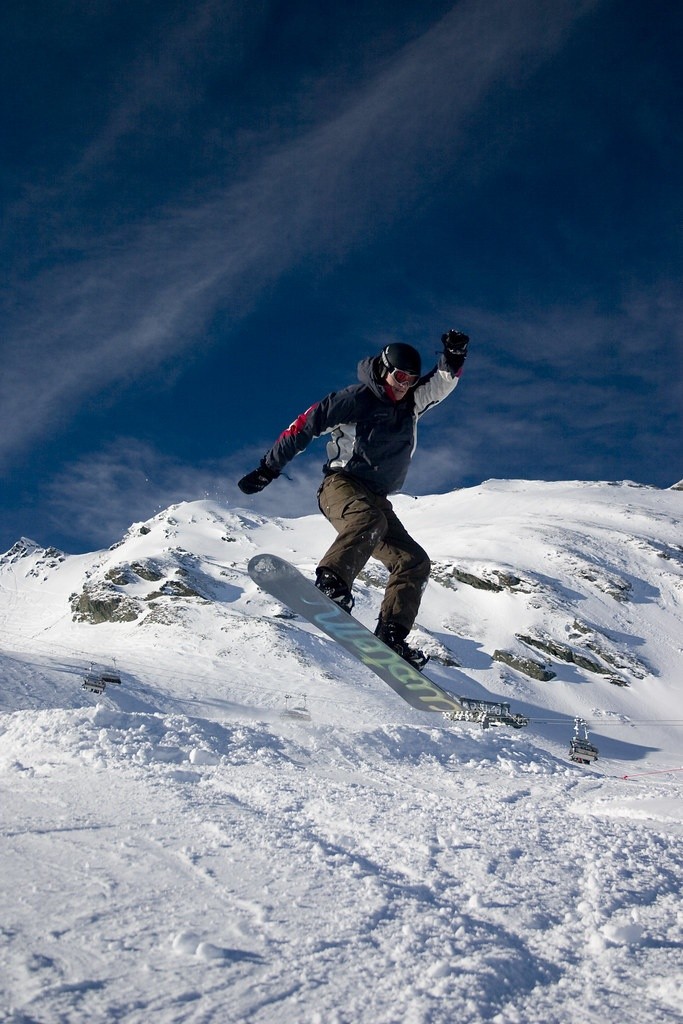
[380,343,421,377]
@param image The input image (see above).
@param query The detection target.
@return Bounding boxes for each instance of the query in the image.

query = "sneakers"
[315,571,355,614]
[374,624,427,672]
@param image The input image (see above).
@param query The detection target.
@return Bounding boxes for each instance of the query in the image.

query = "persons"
[238,328,470,672]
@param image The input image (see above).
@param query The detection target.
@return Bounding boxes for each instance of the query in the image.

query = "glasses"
[390,367,419,387]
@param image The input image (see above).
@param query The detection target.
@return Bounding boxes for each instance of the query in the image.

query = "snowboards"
[248,552,465,713]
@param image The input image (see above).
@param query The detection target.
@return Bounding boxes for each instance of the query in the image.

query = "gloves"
[441,328,470,363]
[237,464,279,495]
